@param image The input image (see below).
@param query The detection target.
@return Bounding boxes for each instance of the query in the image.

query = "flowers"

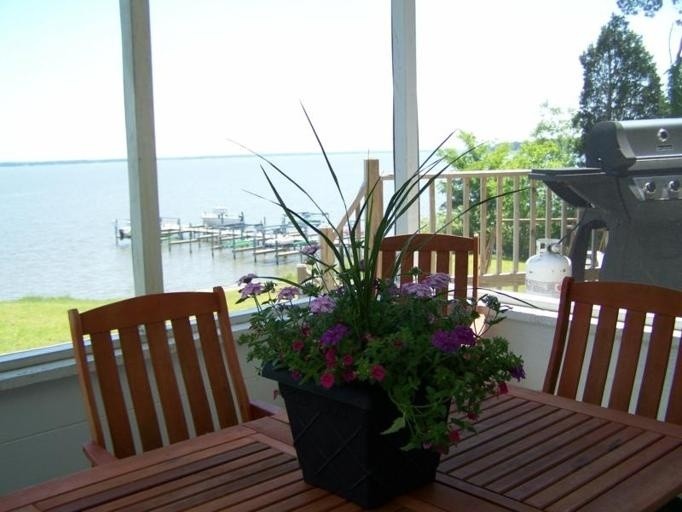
[235,96,527,455]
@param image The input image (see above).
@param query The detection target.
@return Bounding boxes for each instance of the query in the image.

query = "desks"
[2,379,681,511]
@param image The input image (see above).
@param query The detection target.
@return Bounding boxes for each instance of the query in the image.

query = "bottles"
[524,236,572,312]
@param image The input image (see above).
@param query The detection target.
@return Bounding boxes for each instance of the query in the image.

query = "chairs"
[539,274,680,423]
[378,232,481,330]
[66,286,287,468]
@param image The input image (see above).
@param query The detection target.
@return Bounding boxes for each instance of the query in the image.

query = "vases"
[276,381,453,508]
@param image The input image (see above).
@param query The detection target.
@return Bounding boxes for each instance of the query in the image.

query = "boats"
[201,208,244,228]
